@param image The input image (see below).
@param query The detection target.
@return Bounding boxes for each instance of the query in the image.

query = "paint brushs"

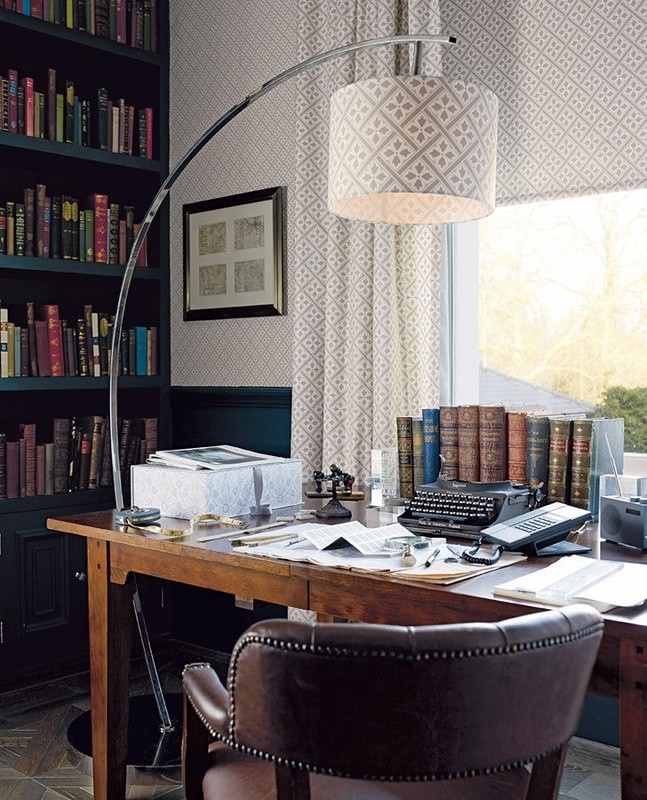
[249,533,299,548]
[232,533,294,544]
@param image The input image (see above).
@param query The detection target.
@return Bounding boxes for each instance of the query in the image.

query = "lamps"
[108,35,497,525]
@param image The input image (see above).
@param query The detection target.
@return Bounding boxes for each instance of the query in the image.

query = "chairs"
[180,604,604,800]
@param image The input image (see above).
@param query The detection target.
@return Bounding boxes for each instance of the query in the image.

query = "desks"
[45,479,647,799]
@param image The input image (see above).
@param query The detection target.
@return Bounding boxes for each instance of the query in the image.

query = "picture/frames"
[155,444,286,471]
[182,186,284,323]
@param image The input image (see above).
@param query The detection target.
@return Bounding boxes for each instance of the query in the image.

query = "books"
[491,554,647,613]
[396,402,624,522]
[0,0,291,497]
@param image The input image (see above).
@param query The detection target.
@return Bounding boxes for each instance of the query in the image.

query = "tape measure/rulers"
[114,505,250,537]
[196,522,286,541]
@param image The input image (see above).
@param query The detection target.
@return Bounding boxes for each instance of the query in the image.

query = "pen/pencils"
[425,549,441,567]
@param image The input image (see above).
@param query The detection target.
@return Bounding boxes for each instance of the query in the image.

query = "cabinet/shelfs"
[0,1,173,720]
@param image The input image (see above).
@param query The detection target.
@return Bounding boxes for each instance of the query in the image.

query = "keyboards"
[403,490,496,523]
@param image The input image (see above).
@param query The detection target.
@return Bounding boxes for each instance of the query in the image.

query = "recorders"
[598,432,647,549]
